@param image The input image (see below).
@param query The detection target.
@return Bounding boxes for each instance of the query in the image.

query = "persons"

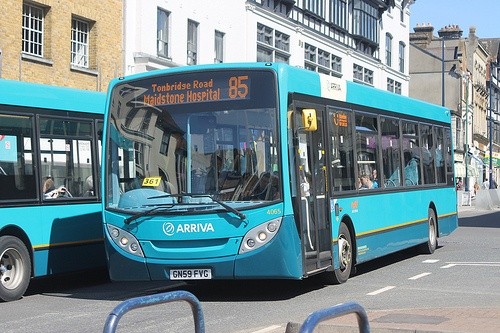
[474,182,480,195]
[458,178,463,189]
[125,163,178,202]
[197,147,271,201]
[483,178,490,192]
[358,164,379,189]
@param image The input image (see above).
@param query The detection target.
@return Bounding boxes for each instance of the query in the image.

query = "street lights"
[462,72,471,192]
[488,60,498,189]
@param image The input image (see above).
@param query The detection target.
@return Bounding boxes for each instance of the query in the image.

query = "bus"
[0,61,459,302]
